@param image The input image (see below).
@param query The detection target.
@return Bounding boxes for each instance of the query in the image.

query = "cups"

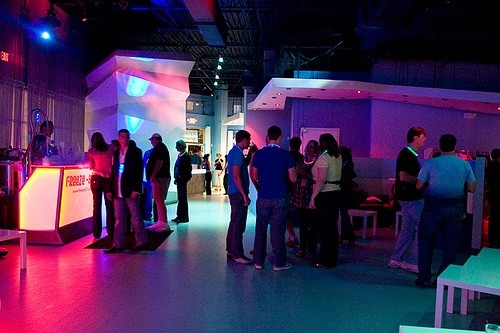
[42,158,50,165]
[249,142,258,152]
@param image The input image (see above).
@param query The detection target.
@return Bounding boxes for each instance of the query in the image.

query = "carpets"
[84,229,174,251]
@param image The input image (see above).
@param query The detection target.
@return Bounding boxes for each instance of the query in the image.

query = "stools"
[396,211,403,234]
[349,209,377,240]
[435,246,500,328]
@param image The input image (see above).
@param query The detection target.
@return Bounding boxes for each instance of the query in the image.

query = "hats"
[148,133,163,141]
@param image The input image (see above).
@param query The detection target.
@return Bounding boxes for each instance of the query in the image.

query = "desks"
[0,230,26,271]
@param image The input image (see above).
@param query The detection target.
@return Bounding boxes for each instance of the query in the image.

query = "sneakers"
[149,220,163,229]
[389,260,403,268]
[156,223,170,232]
[401,262,419,272]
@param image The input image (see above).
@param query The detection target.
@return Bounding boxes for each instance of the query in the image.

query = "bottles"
[189,150,192,155]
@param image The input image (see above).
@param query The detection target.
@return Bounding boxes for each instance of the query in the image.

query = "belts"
[312,180,341,185]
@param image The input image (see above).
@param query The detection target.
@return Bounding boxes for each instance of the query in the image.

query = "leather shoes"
[103,247,124,253]
[235,256,253,264]
[131,242,147,252]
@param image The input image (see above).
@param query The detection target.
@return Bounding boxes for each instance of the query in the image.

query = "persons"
[226,130,254,264]
[28,120,56,163]
[389,127,428,272]
[87,132,115,241]
[219,155,228,195]
[189,146,212,196]
[145,134,171,233]
[249,126,357,271]
[486,149,500,249]
[171,140,192,224]
[415,135,477,287]
[103,128,149,254]
[214,152,224,191]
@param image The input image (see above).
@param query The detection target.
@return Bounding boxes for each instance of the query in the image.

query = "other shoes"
[175,218,188,223]
[296,250,305,257]
[93,236,102,242]
[415,278,427,287]
[286,237,298,247]
[171,216,179,222]
[272,262,293,271]
[254,262,263,269]
[313,260,327,268]
